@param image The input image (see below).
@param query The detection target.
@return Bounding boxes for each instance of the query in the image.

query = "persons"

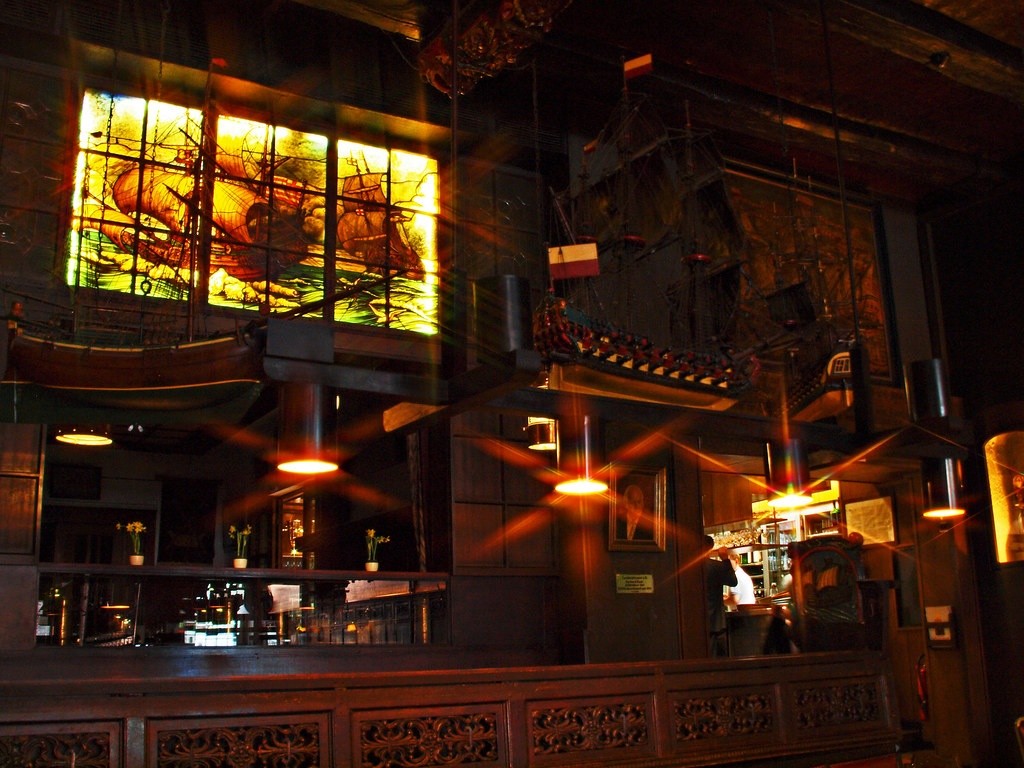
[616,485,654,540]
[762,607,792,655]
[723,554,756,611]
[702,536,737,657]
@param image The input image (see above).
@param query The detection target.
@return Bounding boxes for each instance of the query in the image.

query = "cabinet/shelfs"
[720,545,764,601]
[759,514,840,597]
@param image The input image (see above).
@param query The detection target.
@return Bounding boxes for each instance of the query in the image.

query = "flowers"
[117,522,145,556]
[227,521,253,557]
[366,529,390,560]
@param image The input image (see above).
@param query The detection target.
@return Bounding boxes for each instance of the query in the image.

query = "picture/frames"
[608,464,668,552]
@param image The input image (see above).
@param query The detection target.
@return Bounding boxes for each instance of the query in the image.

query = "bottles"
[707,521,797,596]
[811,506,843,533]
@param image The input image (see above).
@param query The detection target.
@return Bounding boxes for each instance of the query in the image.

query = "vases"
[366,562,379,572]
[130,556,144,566]
[235,559,247,568]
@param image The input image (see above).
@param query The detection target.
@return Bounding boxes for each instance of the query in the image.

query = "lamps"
[523,422,557,450]
[554,419,608,496]
[278,383,339,474]
[913,359,951,424]
[469,273,536,369]
[917,447,969,523]
[55,424,112,446]
